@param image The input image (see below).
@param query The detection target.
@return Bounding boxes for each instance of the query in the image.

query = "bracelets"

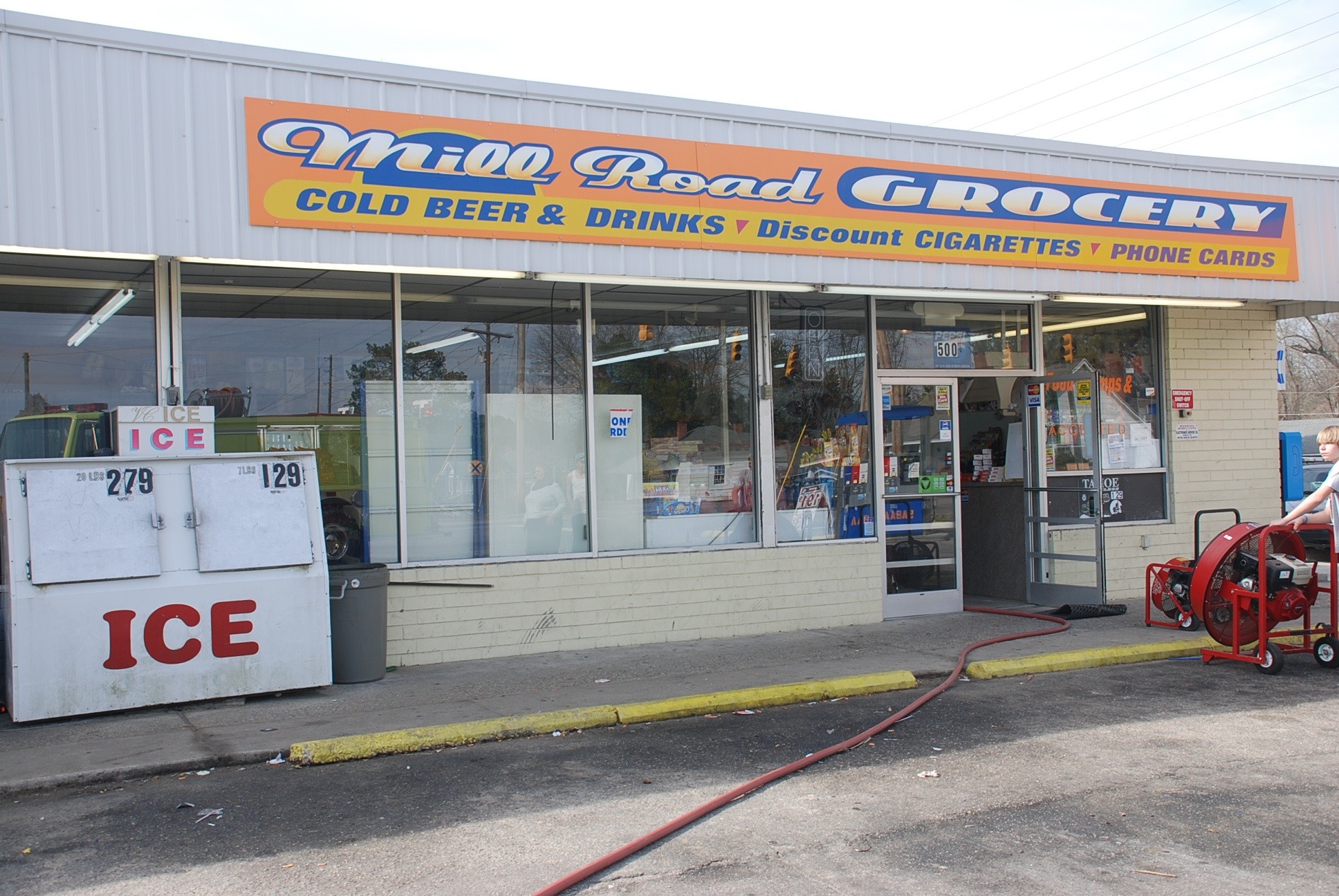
[1303,515,1308,524]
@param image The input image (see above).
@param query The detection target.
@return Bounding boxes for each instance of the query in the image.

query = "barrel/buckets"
[328,562,390,683]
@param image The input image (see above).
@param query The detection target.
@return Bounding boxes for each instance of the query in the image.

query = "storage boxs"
[972,448,1005,483]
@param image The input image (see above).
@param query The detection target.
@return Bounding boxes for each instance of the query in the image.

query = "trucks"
[0,410,370,568]
[1278,418,1339,519]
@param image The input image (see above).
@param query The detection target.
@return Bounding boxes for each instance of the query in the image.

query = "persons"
[1268,426,1339,553]
[522,452,586,554]
[732,452,752,513]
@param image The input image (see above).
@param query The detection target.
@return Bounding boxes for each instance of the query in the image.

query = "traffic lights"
[731,332,744,362]
[1059,333,1072,364]
[638,325,654,341]
[785,352,801,378]
[999,348,1012,370]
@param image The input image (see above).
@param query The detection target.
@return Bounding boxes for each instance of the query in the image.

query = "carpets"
[1040,601,1127,621]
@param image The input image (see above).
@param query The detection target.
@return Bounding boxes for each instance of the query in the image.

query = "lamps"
[0,245,1247,369]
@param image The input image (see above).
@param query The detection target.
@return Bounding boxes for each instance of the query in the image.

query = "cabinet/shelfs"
[483,392,837,557]
